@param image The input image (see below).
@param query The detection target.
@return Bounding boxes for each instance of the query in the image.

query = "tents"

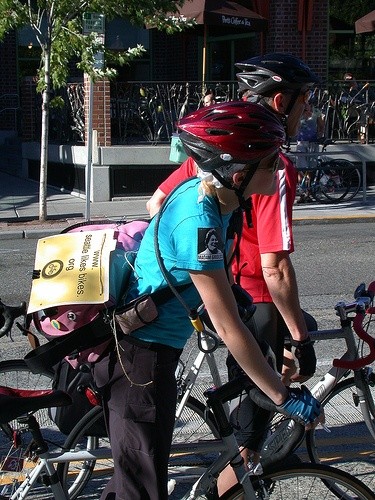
[144,0,270,96]
[355,10,375,35]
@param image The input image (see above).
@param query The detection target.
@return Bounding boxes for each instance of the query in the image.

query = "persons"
[94,102,327,500]
[146,53,367,473]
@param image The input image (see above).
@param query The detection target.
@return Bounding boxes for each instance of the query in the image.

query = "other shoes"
[296,199,316,205]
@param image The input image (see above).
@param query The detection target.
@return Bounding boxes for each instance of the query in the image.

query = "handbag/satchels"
[50,358,111,436]
[31,221,149,371]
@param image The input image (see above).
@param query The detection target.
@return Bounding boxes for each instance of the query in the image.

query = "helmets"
[235,53,321,97]
[176,102,286,171]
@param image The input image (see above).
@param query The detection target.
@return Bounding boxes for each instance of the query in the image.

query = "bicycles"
[281,136,362,205]
[1,279,375,500]
